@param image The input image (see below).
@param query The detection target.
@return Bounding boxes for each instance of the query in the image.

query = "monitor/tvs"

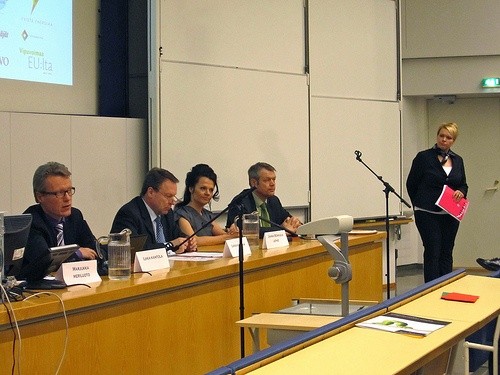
[0,213,33,302]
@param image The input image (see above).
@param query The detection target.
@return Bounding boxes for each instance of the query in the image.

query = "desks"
[0,232,386,375]
[203,268,500,375]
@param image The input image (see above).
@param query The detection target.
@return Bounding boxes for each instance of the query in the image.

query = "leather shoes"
[476,257,500,271]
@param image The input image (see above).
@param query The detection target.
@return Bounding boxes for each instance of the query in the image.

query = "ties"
[260,203,271,227]
[55,223,65,246]
[154,215,165,243]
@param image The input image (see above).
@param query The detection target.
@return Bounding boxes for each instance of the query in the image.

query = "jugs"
[96,228,132,280]
[234,211,260,252]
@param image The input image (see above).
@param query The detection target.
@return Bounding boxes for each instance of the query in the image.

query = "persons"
[227,163,305,236]
[21,162,108,277]
[173,164,240,247]
[111,167,198,254]
[406,122,469,284]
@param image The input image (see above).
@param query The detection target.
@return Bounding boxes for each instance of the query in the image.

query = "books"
[435,186,468,222]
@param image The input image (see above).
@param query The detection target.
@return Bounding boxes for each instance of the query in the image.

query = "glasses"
[39,187,75,198]
[155,188,182,205]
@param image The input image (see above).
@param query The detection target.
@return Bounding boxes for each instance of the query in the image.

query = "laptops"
[93,234,149,276]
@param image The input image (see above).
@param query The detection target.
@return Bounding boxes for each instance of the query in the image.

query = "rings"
[459,194,461,197]
